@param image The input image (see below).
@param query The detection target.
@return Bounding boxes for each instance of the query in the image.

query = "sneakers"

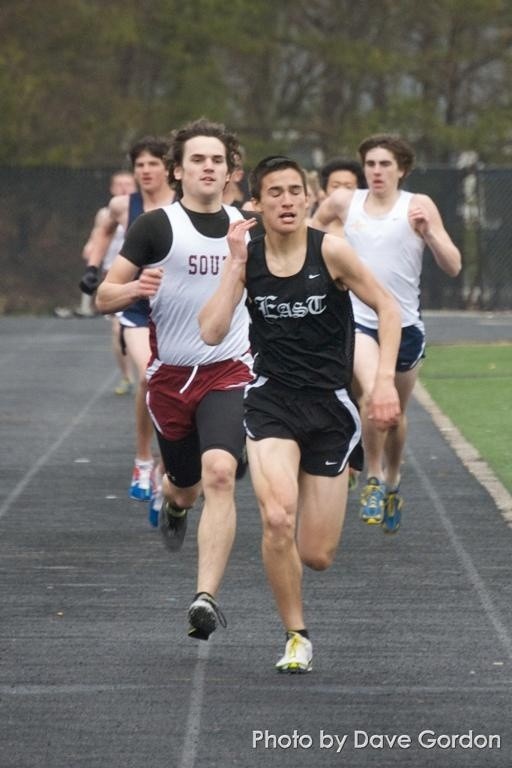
[149,469,163,527]
[348,467,361,493]
[129,458,155,502]
[186,594,219,641]
[381,476,402,534]
[158,500,187,551]
[274,631,313,675]
[358,477,386,525]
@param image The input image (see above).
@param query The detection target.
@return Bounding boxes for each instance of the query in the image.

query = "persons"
[196,153,404,674]
[73,130,463,535]
[92,114,256,643]
